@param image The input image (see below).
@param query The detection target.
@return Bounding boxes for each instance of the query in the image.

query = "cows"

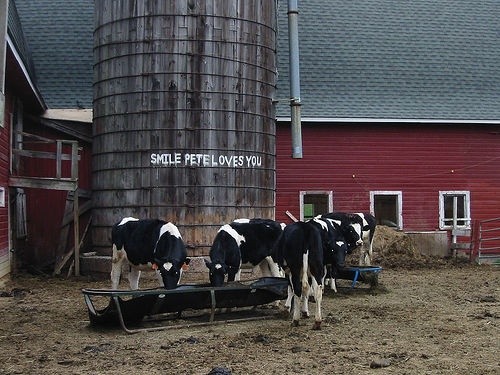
[271,221,327,331]
[204,218,287,314]
[305,217,349,294]
[110,216,190,300]
[332,212,377,266]
